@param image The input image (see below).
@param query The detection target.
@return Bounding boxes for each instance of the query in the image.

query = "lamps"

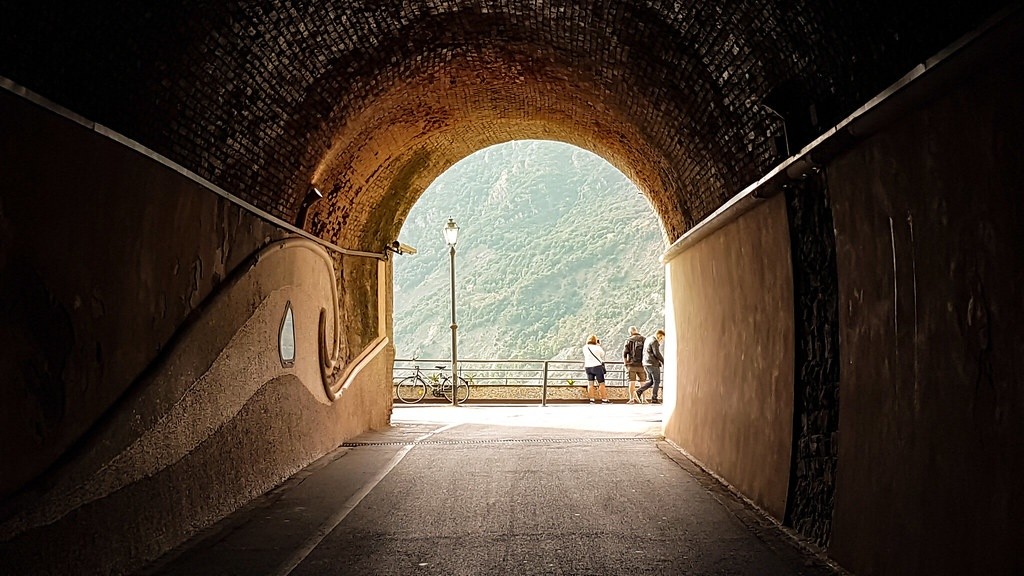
[302,184,323,208]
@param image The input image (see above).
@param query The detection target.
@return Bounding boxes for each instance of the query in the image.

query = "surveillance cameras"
[393,241,417,255]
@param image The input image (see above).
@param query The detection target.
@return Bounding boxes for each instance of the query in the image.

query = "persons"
[622,326,650,404]
[584,334,613,404]
[632,330,665,404]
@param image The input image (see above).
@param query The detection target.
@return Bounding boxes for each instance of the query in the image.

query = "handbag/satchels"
[601,363,606,374]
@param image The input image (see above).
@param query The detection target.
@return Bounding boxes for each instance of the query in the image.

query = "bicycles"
[396,356,470,404]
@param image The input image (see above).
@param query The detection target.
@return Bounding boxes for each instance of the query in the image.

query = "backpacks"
[627,337,645,363]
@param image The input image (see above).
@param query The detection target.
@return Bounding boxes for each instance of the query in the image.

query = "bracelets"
[624,365,628,367]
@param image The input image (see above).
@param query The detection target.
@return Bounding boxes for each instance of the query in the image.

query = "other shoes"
[602,400,613,404]
[635,391,641,403]
[651,399,661,404]
[642,400,649,404]
[626,400,633,404]
[590,400,596,404]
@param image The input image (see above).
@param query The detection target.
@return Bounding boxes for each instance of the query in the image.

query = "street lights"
[442,215,461,406]
